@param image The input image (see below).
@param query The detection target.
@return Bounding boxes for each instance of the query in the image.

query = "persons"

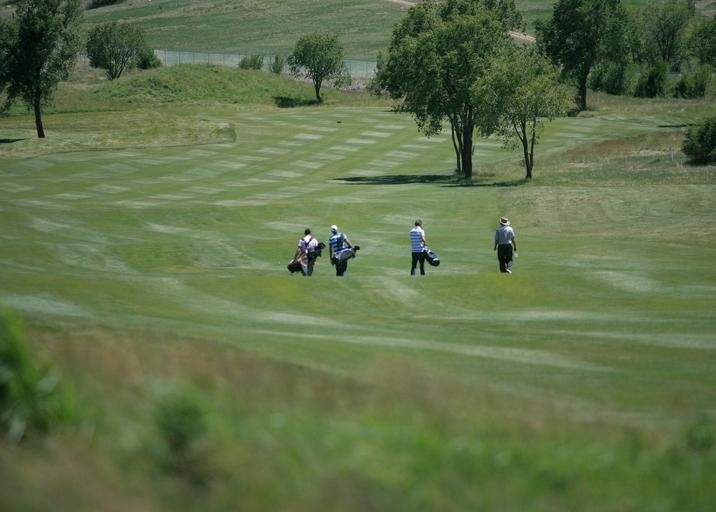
[329,225,353,276]
[408,219,427,276]
[494,217,517,274]
[295,229,318,276]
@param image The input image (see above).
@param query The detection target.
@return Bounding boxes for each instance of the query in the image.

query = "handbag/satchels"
[287,257,304,274]
[423,246,441,267]
[332,246,358,267]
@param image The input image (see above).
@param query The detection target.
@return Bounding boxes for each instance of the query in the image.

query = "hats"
[497,216,512,227]
[330,224,339,233]
